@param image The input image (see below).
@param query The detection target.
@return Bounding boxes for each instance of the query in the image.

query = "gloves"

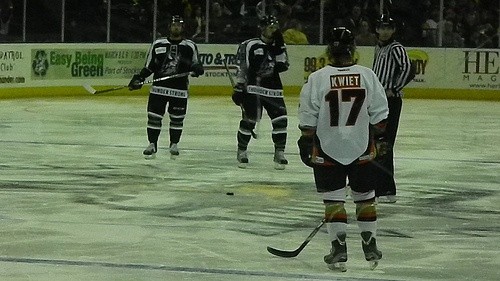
[232,85,245,106]
[271,30,287,52]
[191,64,204,77]
[298,135,321,167]
[127,75,145,90]
[374,134,391,160]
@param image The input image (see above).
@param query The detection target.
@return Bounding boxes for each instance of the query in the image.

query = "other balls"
[226,192,234,196]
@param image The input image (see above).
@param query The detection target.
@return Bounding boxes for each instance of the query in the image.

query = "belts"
[392,92,400,97]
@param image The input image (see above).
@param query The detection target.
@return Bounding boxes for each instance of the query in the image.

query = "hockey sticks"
[82,71,195,95]
[223,56,266,139]
[267,179,351,258]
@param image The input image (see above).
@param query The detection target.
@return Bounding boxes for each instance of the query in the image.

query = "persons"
[295,26,392,264]
[364,13,410,196]
[230,14,291,166]
[128,14,205,158]
[0,0,500,48]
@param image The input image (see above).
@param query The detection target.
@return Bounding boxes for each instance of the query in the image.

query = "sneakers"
[360,231,382,270]
[324,232,348,272]
[143,142,157,158]
[273,149,288,169]
[169,142,180,159]
[236,149,248,167]
[379,189,396,202]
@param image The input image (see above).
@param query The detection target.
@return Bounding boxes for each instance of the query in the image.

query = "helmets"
[168,15,185,29]
[260,15,279,28]
[328,27,354,53]
[376,14,394,25]
